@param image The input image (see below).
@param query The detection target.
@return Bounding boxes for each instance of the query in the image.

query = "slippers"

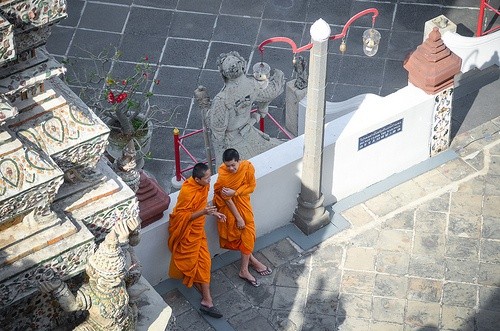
[240,272,260,287]
[199,301,224,318]
[256,266,273,275]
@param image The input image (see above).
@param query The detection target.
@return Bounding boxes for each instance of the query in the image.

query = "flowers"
[63,43,185,161]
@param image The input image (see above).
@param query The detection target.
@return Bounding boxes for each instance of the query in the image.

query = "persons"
[213,148,273,288]
[167,163,227,319]
[193,50,287,174]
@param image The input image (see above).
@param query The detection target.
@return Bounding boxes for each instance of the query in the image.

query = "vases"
[104,110,153,160]
